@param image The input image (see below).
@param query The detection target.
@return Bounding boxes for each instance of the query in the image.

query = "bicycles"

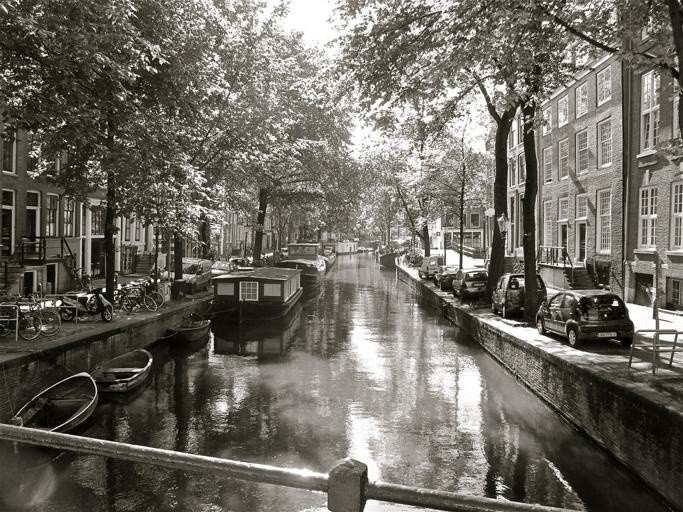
[0,272,166,343]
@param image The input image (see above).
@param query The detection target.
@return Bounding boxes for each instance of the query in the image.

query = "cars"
[162,255,253,296]
[418,254,639,348]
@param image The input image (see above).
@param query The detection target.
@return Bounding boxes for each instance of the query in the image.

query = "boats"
[88,349,159,392]
[207,304,305,363]
[207,263,306,317]
[315,243,338,270]
[275,240,328,293]
[7,369,103,452]
[164,316,214,342]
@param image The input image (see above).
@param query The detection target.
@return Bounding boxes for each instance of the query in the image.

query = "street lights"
[495,212,510,273]
[243,222,250,259]
[153,219,160,293]
[441,222,449,267]
[411,226,417,255]
